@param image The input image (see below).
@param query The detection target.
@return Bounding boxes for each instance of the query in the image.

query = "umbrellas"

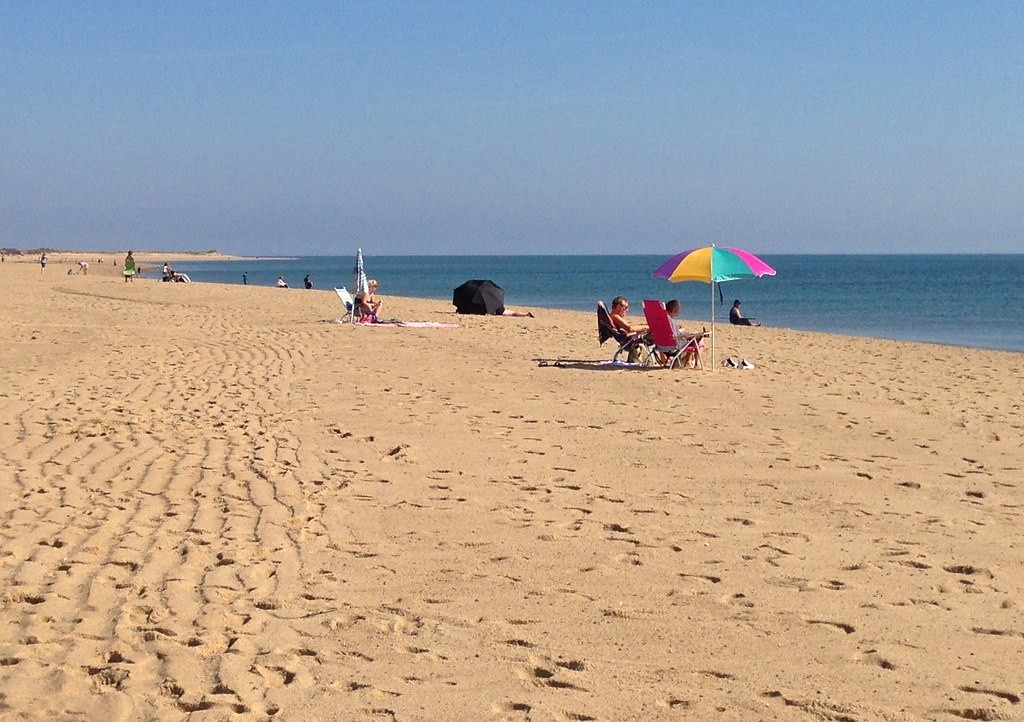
[653,243,776,370]
[452,279,505,314]
[350,247,369,324]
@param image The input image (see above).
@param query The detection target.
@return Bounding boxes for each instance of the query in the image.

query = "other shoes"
[371,318,383,323]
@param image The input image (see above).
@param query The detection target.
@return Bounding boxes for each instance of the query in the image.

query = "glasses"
[621,304,630,311]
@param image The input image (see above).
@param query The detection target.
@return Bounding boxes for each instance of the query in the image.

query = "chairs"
[333,286,370,323]
[597,299,662,366]
[641,299,710,370]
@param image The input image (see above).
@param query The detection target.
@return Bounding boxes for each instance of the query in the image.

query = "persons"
[278,277,291,289]
[242,272,248,285]
[656,299,706,370]
[729,300,762,327]
[495,307,535,318]
[610,297,649,337]
[304,275,314,289]
[355,280,384,324]
[41,250,191,284]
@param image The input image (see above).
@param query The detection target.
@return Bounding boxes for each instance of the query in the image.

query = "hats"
[734,300,742,304]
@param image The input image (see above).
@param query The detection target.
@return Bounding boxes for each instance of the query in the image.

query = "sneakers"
[738,358,755,370]
[721,357,738,369]
